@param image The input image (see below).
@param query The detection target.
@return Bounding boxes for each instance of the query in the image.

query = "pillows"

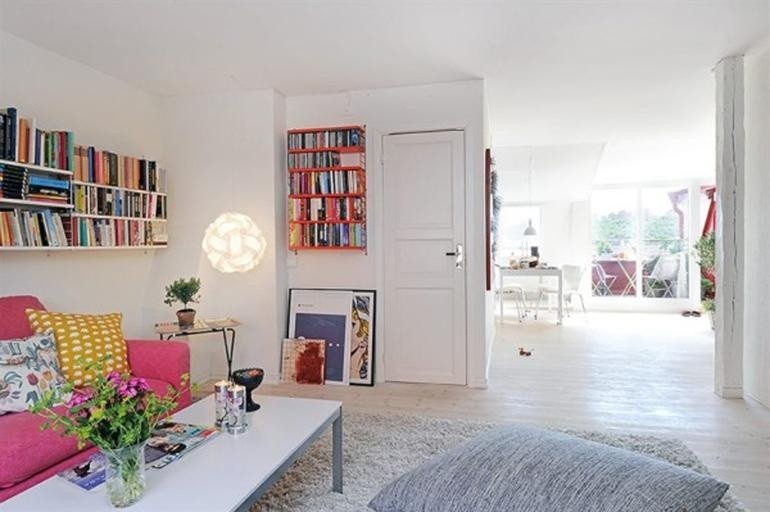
[0,332,74,416]
[368,419,730,512]
[23,307,131,400]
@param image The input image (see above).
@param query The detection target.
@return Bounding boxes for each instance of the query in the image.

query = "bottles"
[227,386,247,435]
[214,379,234,430]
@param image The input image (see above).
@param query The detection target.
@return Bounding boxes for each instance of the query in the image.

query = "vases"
[100,445,145,509]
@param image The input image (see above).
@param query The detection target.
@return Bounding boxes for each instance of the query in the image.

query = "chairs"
[491,230,717,333]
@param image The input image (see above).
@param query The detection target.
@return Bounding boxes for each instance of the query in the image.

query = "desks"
[156,322,238,387]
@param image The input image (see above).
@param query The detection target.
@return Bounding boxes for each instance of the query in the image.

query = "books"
[288,131,360,247]
[0,107,169,245]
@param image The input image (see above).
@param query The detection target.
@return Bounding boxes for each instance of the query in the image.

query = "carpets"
[250,407,748,512]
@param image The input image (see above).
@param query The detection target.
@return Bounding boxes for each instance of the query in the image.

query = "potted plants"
[163,278,200,324]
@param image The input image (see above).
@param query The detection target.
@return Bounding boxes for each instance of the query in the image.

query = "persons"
[146,435,187,454]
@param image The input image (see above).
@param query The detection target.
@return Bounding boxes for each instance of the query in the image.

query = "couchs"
[0,295,191,500]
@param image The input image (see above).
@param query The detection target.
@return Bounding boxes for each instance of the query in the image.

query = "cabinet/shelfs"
[72,180,169,248]
[2,157,75,254]
[285,124,367,252]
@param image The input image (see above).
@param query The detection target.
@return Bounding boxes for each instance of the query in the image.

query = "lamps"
[524,146,537,236]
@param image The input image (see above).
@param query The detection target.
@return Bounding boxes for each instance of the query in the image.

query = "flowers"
[24,353,200,505]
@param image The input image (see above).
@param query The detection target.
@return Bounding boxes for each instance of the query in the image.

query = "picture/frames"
[289,290,354,388]
[286,288,378,387]
